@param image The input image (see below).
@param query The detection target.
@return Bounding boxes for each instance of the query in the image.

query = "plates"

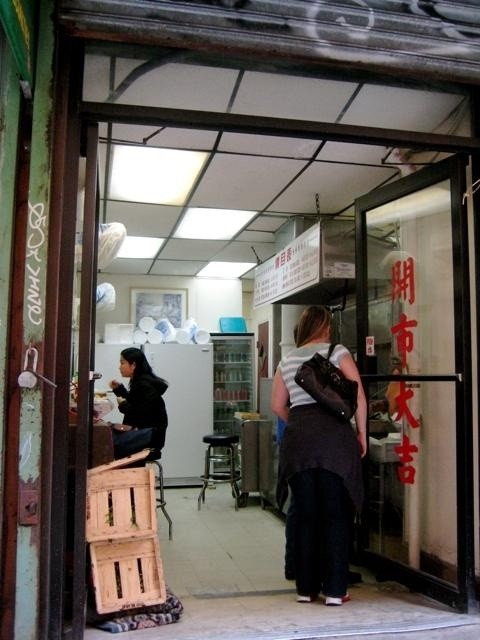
[131,315,210,344]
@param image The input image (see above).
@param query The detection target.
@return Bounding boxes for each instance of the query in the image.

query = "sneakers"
[348,570,361,585]
[296,594,319,602]
[325,590,349,605]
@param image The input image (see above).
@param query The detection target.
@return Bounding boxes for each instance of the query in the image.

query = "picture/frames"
[127,285,190,333]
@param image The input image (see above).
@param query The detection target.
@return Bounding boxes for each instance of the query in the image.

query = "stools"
[196,434,243,512]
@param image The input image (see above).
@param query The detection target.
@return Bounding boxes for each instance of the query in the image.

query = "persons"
[270,304,369,606]
[272,323,364,585]
[367,350,404,425]
[109,347,169,511]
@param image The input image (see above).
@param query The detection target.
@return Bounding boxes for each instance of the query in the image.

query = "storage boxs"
[84,446,167,616]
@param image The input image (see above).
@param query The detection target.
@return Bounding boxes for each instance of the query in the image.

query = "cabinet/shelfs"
[231,374,280,517]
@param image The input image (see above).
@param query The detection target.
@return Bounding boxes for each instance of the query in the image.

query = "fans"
[98,221,127,273]
[95,281,118,317]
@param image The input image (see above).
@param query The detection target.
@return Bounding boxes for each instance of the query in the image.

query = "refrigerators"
[210,332,259,466]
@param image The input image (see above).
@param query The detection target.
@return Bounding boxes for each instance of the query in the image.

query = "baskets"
[368,419,388,433]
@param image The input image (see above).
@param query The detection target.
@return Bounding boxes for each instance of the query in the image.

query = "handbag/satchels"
[294,342,359,423]
